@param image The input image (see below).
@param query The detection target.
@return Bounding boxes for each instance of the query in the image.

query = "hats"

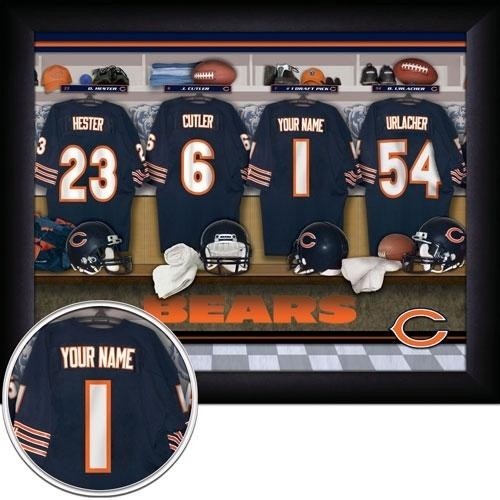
[301,68,325,85]
[41,64,72,94]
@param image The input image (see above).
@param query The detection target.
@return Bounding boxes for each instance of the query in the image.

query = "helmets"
[200,219,252,274]
[65,221,133,275]
[400,216,467,273]
[288,220,348,275]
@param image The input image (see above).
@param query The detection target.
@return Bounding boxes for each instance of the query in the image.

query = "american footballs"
[392,58,438,84]
[192,61,236,84]
[377,234,415,262]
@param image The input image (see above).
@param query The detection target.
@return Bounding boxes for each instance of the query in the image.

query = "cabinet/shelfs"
[33,53,467,343]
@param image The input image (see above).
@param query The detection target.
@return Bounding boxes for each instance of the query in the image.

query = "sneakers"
[112,64,129,84]
[360,63,378,85]
[94,66,111,83]
[264,64,298,84]
[378,64,396,84]
